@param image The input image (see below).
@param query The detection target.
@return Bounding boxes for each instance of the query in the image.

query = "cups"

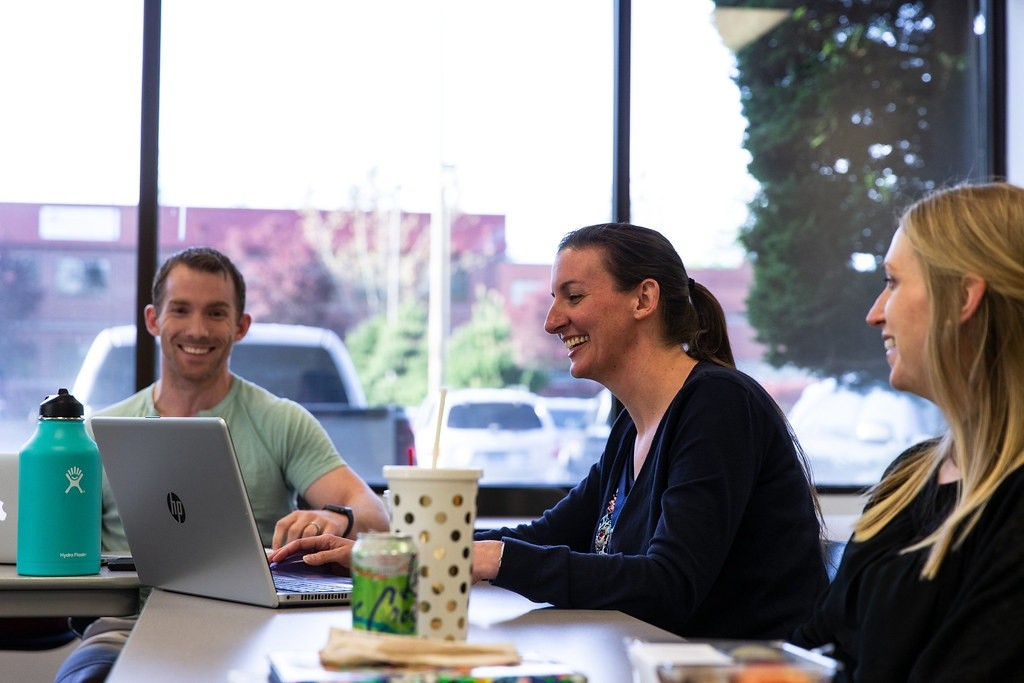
[382,466,484,642]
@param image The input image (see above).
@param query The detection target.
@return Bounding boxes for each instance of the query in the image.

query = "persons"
[818,182,1024,683]
[53,246,391,683]
[268,222,830,650]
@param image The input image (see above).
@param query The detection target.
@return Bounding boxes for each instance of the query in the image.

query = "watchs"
[321,503,354,538]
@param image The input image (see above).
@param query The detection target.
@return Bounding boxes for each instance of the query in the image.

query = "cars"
[71,324,363,432]
[404,388,614,476]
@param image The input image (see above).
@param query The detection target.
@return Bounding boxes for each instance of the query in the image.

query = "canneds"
[350,531,420,636]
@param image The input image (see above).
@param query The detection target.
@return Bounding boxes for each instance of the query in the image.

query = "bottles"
[16,390,103,574]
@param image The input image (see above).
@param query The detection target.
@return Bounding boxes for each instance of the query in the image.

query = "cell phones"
[108,558,137,571]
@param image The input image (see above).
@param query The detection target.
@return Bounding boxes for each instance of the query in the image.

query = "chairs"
[819,539,847,584]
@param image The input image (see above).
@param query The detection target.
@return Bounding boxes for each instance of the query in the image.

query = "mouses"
[269,549,350,577]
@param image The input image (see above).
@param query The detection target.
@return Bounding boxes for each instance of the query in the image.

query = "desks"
[0,563,151,617]
[105,580,690,683]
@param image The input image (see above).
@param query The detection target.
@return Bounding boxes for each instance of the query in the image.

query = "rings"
[310,522,320,532]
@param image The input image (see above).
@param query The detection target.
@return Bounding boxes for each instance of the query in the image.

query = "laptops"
[92,416,355,608]
[0,451,19,565]
